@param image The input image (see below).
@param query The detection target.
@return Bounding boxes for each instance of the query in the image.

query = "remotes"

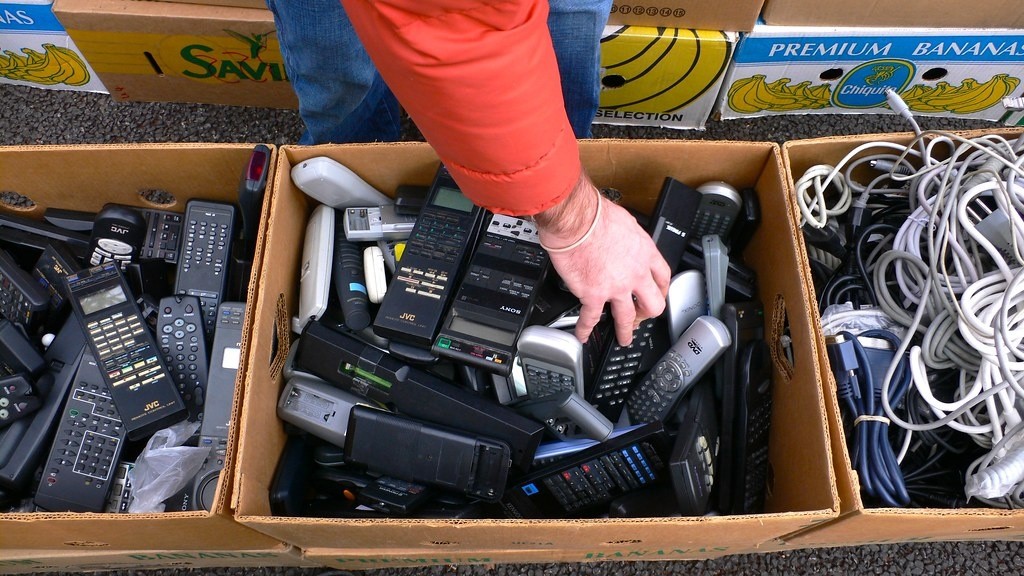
[0,143,270,516]
[271,156,774,522]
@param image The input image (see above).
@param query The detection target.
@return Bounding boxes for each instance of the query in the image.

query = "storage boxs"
[1,0,1024,569]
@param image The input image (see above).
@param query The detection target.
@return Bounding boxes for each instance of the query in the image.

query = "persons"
[265,0,671,347]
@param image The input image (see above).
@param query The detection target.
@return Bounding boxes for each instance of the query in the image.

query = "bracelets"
[539,185,602,253]
